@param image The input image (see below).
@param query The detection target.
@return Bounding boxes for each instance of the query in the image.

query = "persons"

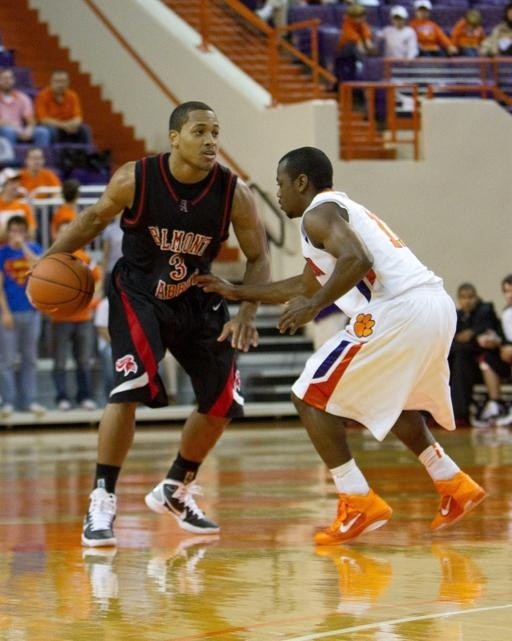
[447,273,511,425]
[24,102,273,548]
[337,1,512,59]
[192,145,488,544]
[1,69,124,417]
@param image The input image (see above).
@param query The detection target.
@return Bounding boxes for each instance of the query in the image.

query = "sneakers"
[429,470,490,532]
[0,397,99,419]
[145,534,221,597]
[468,399,512,428]
[80,488,118,548]
[431,539,490,609]
[314,545,391,608]
[80,546,122,603]
[313,486,393,547]
[143,477,222,536]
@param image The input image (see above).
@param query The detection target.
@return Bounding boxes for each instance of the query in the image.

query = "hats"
[0,166,26,188]
[346,0,433,21]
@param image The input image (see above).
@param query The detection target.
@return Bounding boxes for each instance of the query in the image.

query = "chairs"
[235,0,512,121]
[1,37,107,205]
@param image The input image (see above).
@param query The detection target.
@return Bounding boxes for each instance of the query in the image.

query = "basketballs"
[27,253,95,318]
